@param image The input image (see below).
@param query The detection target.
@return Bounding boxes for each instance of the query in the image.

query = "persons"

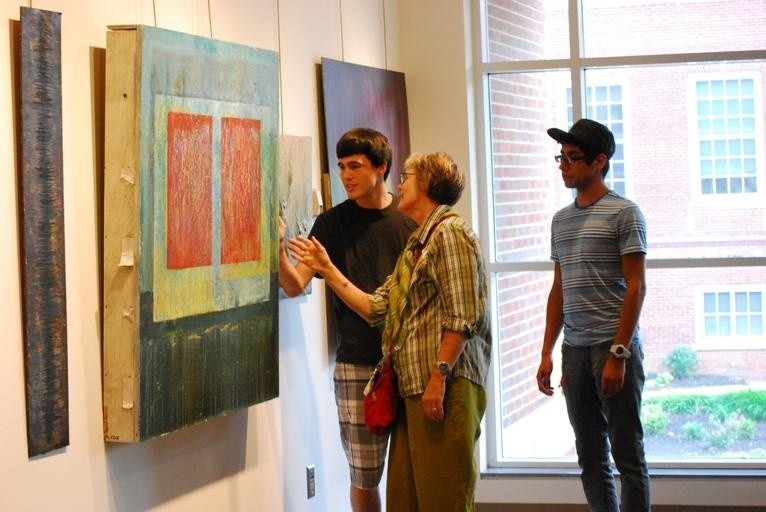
[286,150,498,512]
[272,128,421,511]
[531,119,665,512]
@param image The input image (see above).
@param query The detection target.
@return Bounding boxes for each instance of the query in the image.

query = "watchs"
[608,343,632,358]
[433,359,455,375]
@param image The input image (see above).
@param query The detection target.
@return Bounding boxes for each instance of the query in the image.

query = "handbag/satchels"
[365,352,397,435]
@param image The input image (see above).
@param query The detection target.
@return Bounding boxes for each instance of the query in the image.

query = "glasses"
[399,171,419,184]
[556,155,587,164]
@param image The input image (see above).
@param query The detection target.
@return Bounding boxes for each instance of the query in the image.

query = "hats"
[547,120,616,156]
